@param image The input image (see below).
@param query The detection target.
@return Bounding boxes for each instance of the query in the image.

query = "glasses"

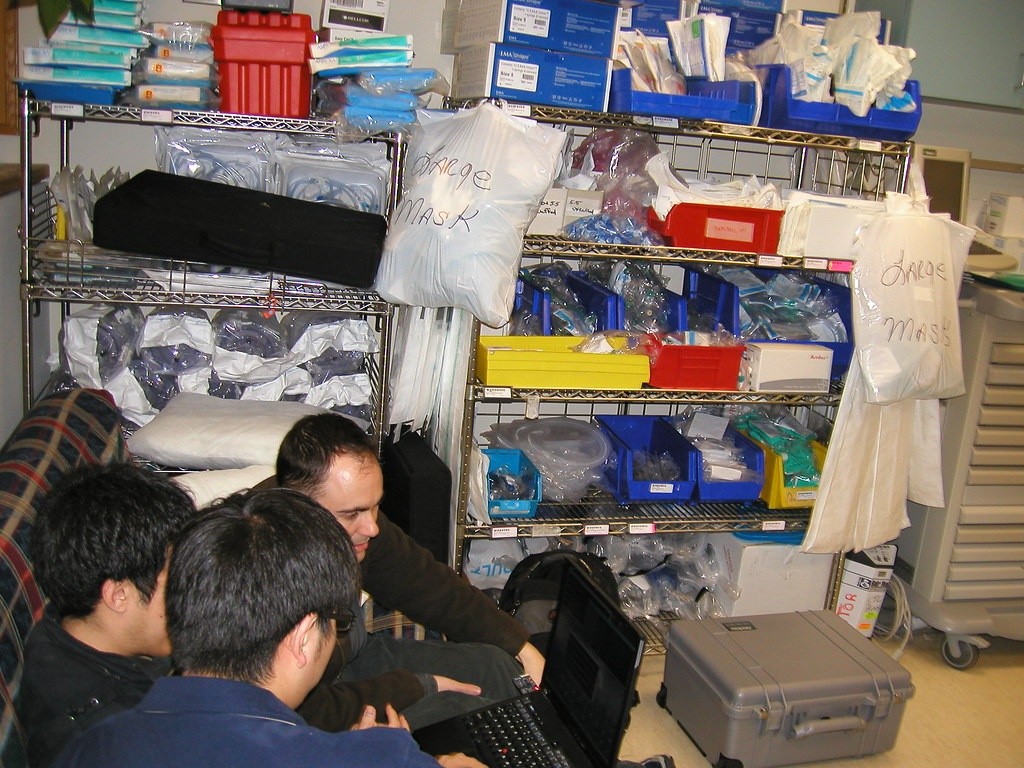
[298,607,356,631]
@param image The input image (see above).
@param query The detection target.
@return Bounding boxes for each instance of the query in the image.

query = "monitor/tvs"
[917,144,972,228]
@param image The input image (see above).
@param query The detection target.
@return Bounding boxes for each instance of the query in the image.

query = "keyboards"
[963,240,1020,274]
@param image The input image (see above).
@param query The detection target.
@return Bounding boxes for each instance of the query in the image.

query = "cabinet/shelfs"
[873,274,1024,669]
[21,88,408,463]
[446,98,926,614]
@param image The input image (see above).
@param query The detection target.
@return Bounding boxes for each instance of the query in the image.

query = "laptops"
[414,553,647,768]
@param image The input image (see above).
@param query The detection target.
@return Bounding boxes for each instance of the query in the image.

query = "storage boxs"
[320,0,392,31]
[710,531,834,615]
[9,0,215,111]
[620,0,687,58]
[650,330,747,393]
[663,414,765,506]
[454,0,624,61]
[595,412,696,506]
[451,44,616,112]
[613,59,756,124]
[746,340,835,395]
[660,613,916,768]
[836,543,899,640]
[752,63,922,142]
[985,191,1024,240]
[689,0,786,12]
[644,201,785,254]
[737,424,831,511]
[209,9,317,113]
[476,336,650,390]
[787,9,893,48]
[480,446,545,520]
[440,5,460,55]
[691,3,785,52]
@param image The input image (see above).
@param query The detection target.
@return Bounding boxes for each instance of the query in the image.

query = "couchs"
[0,386,446,768]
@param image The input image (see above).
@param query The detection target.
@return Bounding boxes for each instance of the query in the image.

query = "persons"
[243,412,546,734]
[15,463,443,768]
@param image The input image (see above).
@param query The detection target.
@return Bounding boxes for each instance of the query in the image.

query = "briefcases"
[656,608,915,768]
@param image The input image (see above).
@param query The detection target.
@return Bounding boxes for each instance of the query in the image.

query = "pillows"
[172,463,278,510]
[122,390,372,469]
